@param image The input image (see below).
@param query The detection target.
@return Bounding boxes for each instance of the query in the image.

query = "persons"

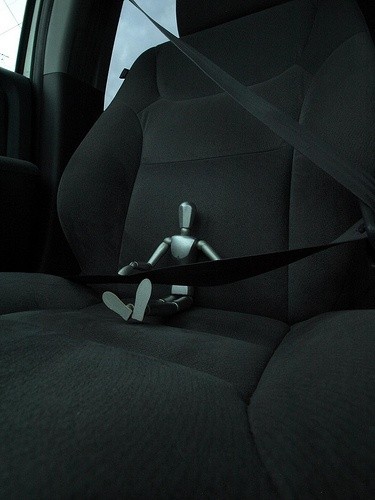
[100,200,225,325]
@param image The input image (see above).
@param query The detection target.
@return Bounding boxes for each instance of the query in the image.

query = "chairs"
[0,0,375,500]
